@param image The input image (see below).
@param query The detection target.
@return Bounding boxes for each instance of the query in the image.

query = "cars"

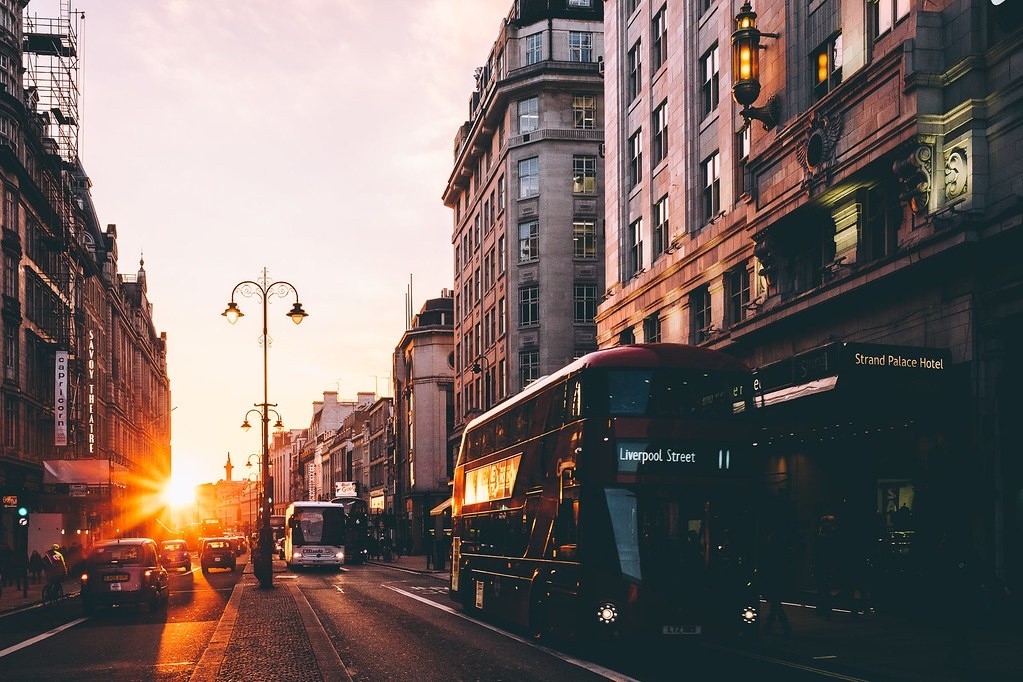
[83,537,171,617]
[159,538,191,572]
[219,535,286,560]
[199,539,238,572]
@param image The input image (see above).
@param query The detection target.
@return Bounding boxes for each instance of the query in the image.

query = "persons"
[352,483,357,495]
[368,535,451,562]
[0,542,83,605]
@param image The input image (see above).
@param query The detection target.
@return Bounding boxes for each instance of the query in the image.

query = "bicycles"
[361,529,400,563]
[41,573,67,607]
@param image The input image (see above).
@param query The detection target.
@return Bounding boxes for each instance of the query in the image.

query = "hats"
[52,544,59,549]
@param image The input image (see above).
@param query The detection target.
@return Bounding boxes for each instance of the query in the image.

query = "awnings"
[430,497,453,516]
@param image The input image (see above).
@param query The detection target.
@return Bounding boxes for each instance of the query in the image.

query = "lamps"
[728,3,782,132]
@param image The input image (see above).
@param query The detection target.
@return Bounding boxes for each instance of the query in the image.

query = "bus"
[269,514,286,550]
[284,500,350,572]
[328,496,372,559]
[450,341,772,661]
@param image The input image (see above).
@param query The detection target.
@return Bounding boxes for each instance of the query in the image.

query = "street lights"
[221,268,309,587]
[241,402,285,519]
[474,354,490,413]
